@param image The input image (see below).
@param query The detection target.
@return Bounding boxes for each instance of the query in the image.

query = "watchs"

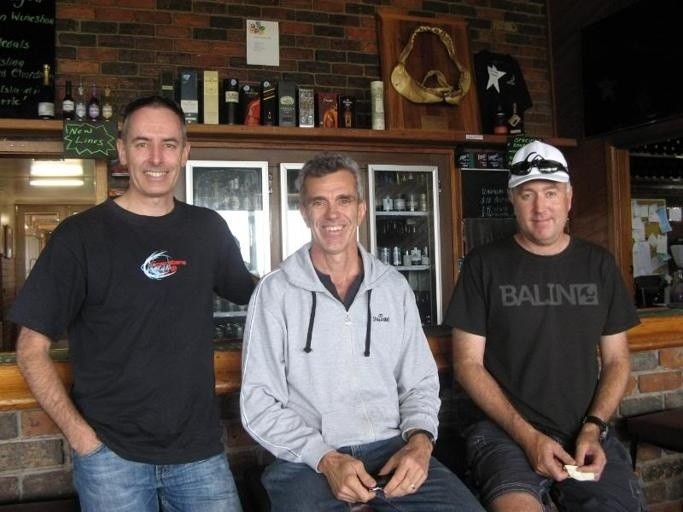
[581,412,610,443]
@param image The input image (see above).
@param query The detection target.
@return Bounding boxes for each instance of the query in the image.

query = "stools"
[619,406,682,472]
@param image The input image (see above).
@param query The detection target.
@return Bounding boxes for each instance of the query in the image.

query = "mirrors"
[0,140,109,368]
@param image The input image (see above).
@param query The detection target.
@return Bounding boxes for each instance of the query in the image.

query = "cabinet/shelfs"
[171,121,460,347]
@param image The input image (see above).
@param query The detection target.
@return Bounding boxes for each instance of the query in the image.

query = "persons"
[238,151,489,511]
[6,95,257,511]
[442,137,643,511]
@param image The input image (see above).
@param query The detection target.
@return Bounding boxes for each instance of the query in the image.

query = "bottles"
[374,171,427,211]
[75,82,86,121]
[192,169,261,210]
[61,80,75,119]
[36,63,56,119]
[376,216,428,265]
[101,84,114,122]
[87,83,101,122]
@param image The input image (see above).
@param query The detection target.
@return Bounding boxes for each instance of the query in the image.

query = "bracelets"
[407,429,435,448]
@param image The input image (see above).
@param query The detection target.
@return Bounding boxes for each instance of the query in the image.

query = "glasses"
[506,159,569,181]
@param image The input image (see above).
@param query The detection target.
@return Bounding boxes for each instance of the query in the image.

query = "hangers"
[487,38,501,54]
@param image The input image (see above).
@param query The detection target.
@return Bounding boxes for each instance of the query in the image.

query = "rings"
[409,482,417,490]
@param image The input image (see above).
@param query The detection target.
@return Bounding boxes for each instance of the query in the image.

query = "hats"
[507,138,570,187]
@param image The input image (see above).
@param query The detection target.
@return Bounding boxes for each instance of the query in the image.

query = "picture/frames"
[3,224,15,259]
